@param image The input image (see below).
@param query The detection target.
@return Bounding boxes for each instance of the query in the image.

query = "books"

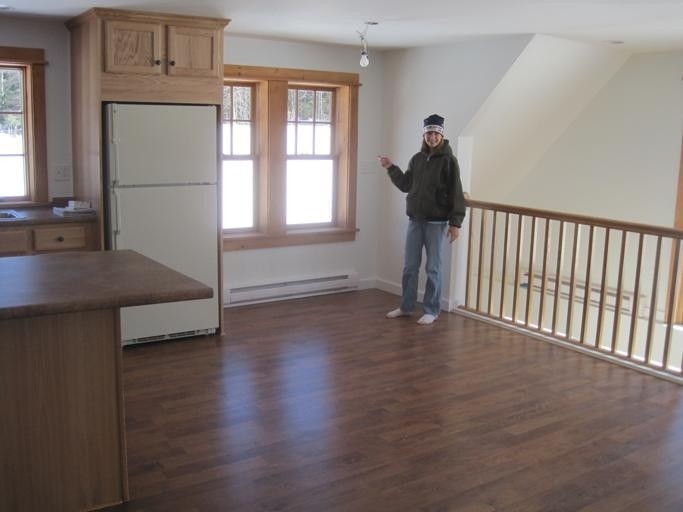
[52,206,97,219]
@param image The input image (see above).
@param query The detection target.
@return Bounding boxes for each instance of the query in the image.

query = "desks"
[1,247,213,510]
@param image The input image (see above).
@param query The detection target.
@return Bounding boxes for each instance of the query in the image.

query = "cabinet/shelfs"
[1,203,98,258]
[71,18,233,87]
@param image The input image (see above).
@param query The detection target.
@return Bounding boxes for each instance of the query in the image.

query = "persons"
[375,111,467,325]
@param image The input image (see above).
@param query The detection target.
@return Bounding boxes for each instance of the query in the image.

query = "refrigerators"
[103,102,221,350]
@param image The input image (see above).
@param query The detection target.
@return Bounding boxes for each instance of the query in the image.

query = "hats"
[423,114,445,137]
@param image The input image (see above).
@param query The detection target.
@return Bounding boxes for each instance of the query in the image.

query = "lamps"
[357,25,371,68]
